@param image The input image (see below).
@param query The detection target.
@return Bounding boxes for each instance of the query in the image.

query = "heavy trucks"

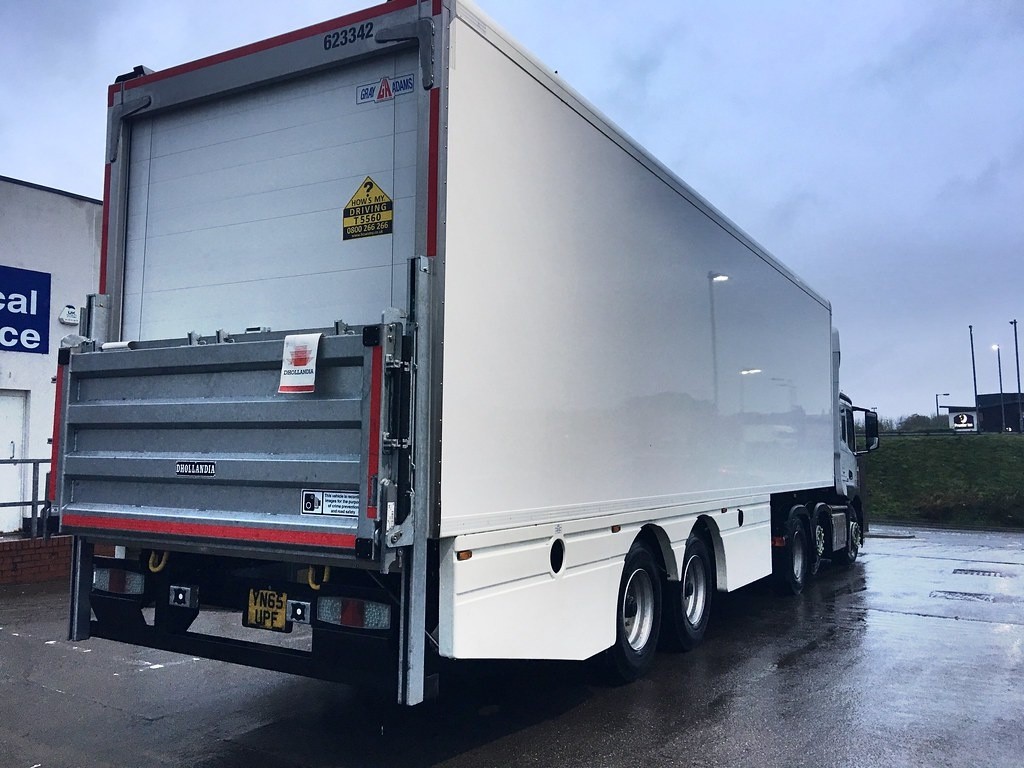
[47,0,880,707]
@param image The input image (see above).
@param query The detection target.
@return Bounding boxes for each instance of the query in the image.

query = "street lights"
[1008,319,1024,432]
[992,344,1007,434]
[936,393,950,417]
